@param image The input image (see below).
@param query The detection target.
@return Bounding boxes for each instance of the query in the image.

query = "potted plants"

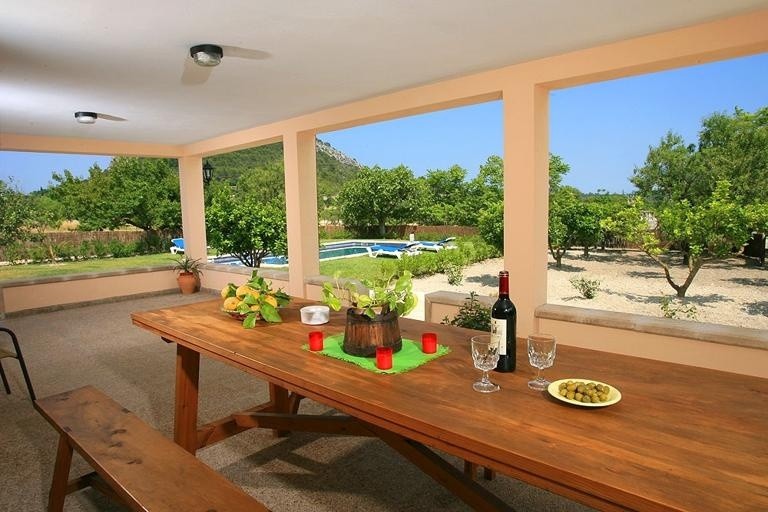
[168,257,204,294]
[321,265,415,357]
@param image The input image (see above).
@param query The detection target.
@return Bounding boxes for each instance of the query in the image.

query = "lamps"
[188,44,223,68]
[75,112,97,124]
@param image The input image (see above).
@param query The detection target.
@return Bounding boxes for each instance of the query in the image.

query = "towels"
[301,330,450,377]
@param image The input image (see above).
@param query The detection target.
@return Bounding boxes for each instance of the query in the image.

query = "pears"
[222,285,277,311]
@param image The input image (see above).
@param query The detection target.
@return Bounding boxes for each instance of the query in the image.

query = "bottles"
[490,270,517,373]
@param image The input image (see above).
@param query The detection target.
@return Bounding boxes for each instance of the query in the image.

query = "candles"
[422,333,438,354]
[376,346,394,369]
[307,331,323,350]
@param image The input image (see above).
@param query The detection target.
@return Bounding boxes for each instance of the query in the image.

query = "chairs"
[367,236,457,260]
[170,236,211,254]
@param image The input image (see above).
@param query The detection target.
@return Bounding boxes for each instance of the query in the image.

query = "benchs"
[30,384,280,511]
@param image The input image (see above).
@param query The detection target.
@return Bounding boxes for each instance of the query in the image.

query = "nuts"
[559,380,610,403]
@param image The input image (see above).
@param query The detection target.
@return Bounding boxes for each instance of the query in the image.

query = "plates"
[548,378,623,408]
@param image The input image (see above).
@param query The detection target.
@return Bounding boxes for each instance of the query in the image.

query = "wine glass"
[471,333,501,393]
[527,332,557,392]
